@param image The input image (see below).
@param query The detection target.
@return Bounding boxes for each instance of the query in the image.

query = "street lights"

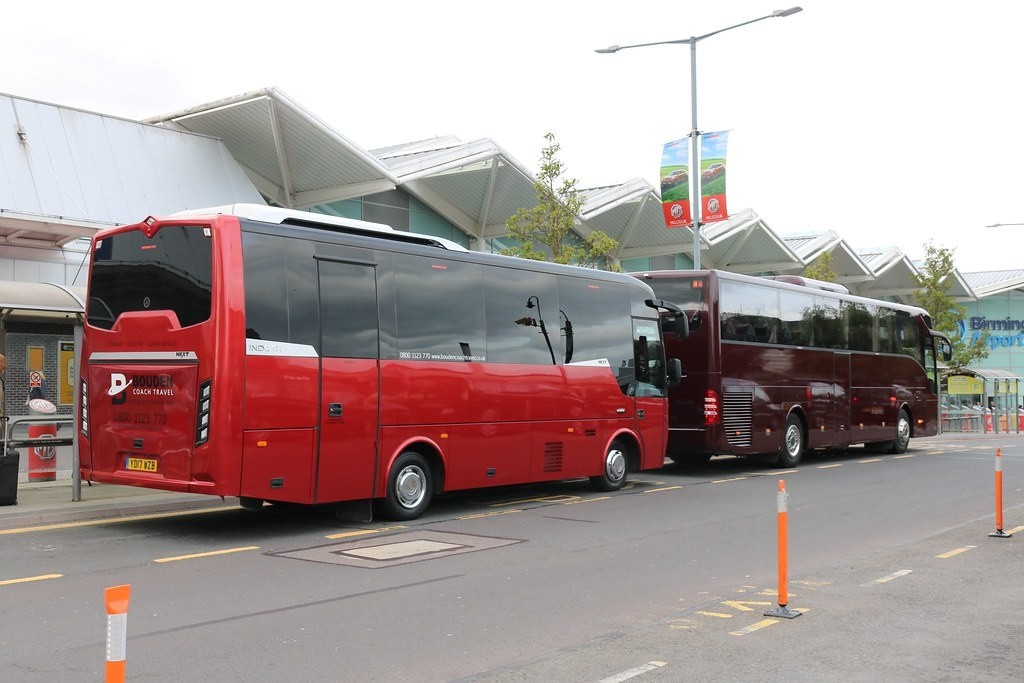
[593,3,804,259]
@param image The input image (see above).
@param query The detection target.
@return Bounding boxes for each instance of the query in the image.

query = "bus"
[615,270,956,470]
[78,205,691,523]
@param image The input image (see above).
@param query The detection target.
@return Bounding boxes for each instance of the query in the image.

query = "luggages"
[0,416,20,507]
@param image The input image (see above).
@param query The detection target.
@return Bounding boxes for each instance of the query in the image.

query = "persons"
[0,354,7,415]
[25,372,46,406]
[975,401,1024,415]
